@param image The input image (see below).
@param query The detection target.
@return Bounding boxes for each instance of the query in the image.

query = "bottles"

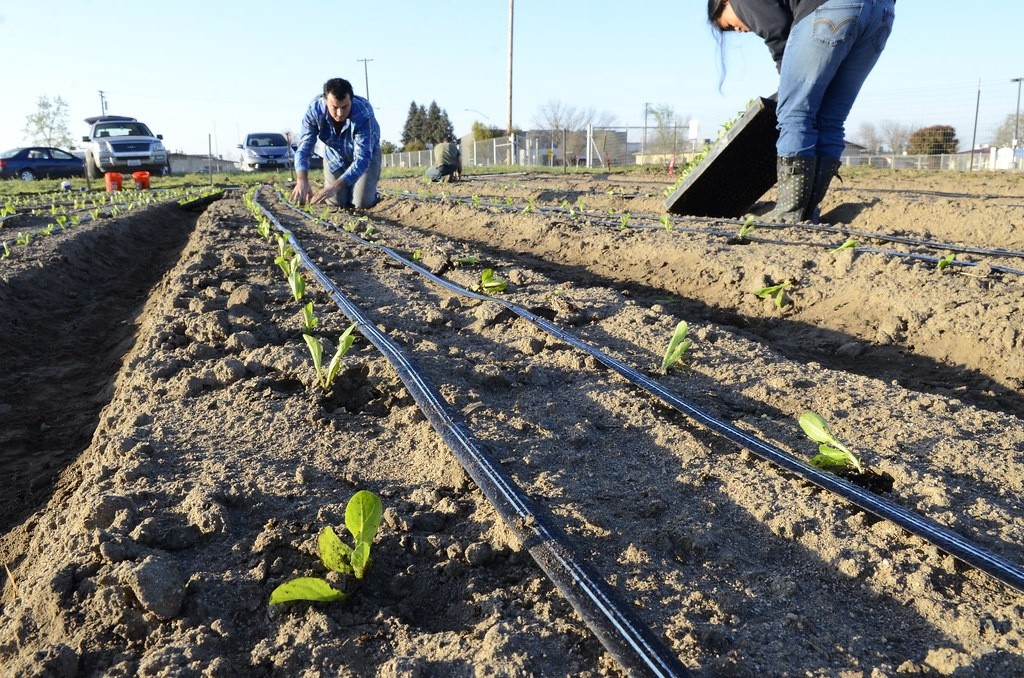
[80,187,85,192]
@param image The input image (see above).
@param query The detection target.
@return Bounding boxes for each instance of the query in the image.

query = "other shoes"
[443,175,455,184]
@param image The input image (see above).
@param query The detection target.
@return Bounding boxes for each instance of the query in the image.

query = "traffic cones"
[667,161,674,174]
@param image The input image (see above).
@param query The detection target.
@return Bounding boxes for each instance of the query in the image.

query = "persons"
[707,0,898,225]
[288,77,382,208]
[425,136,462,182]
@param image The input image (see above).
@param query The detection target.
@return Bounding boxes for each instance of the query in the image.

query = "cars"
[237,133,298,172]
[0,147,84,181]
[309,152,323,169]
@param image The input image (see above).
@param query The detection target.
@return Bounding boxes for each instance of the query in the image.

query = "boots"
[807,153,843,218]
[744,156,817,222]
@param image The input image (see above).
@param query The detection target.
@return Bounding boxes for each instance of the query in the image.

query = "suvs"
[82,116,170,179]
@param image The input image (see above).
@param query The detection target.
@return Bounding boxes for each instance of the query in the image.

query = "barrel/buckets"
[104,172,122,192]
[61,182,71,190]
[132,171,150,190]
